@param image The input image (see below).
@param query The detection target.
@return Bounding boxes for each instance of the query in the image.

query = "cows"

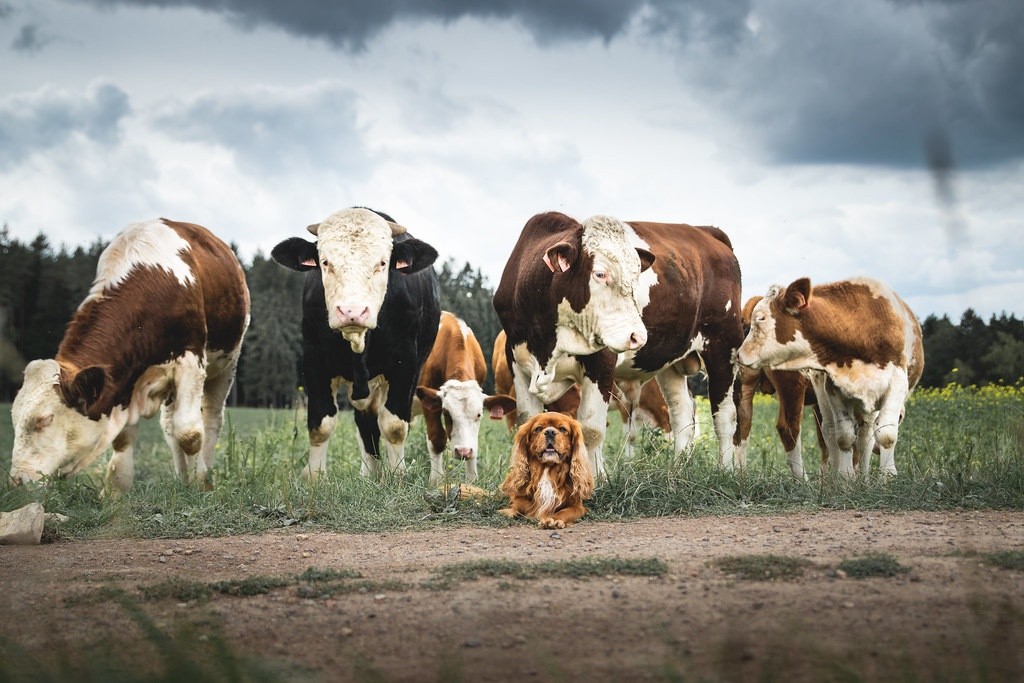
[491,210,748,471]
[409,311,516,484]
[7,218,252,502]
[732,274,926,489]
[271,207,442,484]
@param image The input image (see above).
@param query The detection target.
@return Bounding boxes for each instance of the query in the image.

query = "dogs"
[497,411,598,531]
